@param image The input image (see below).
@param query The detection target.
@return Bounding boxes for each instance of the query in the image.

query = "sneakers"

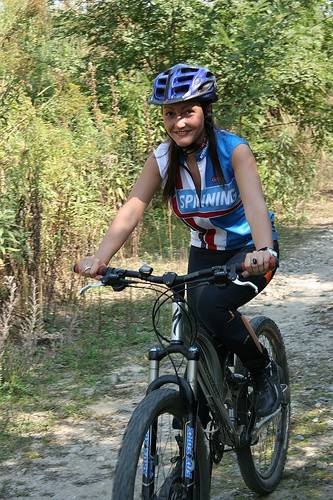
[250,361,285,416]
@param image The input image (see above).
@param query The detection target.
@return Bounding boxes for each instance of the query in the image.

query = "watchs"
[257,247,277,257]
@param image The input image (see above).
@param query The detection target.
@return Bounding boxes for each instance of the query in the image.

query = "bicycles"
[73,256,291,500]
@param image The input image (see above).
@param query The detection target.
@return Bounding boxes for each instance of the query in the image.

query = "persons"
[76,63,285,443]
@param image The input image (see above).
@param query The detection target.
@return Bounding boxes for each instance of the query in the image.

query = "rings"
[250,258,257,264]
[83,265,90,272]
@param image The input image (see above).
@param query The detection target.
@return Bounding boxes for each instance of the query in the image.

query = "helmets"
[148,63,218,105]
